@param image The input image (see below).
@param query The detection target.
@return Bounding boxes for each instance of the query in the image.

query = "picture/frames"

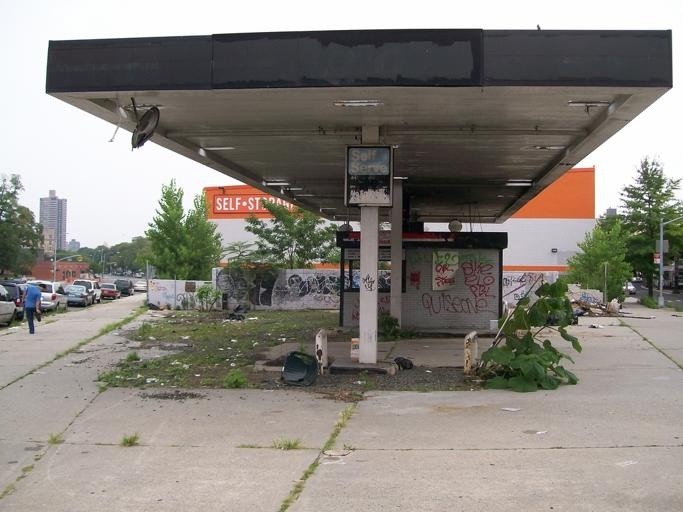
[344,143,394,209]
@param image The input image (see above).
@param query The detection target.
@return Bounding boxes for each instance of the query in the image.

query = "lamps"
[127,95,159,148]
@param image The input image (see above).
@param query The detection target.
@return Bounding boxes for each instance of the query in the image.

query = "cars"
[101,279,148,299]
[66,279,102,306]
[0,280,70,327]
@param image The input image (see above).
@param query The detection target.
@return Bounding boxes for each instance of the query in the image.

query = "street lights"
[52,249,82,281]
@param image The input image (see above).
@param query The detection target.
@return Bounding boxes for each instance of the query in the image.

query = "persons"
[21,283,46,335]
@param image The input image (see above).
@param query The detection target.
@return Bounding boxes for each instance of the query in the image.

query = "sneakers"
[35,313,41,322]
[30,330,34,333]
